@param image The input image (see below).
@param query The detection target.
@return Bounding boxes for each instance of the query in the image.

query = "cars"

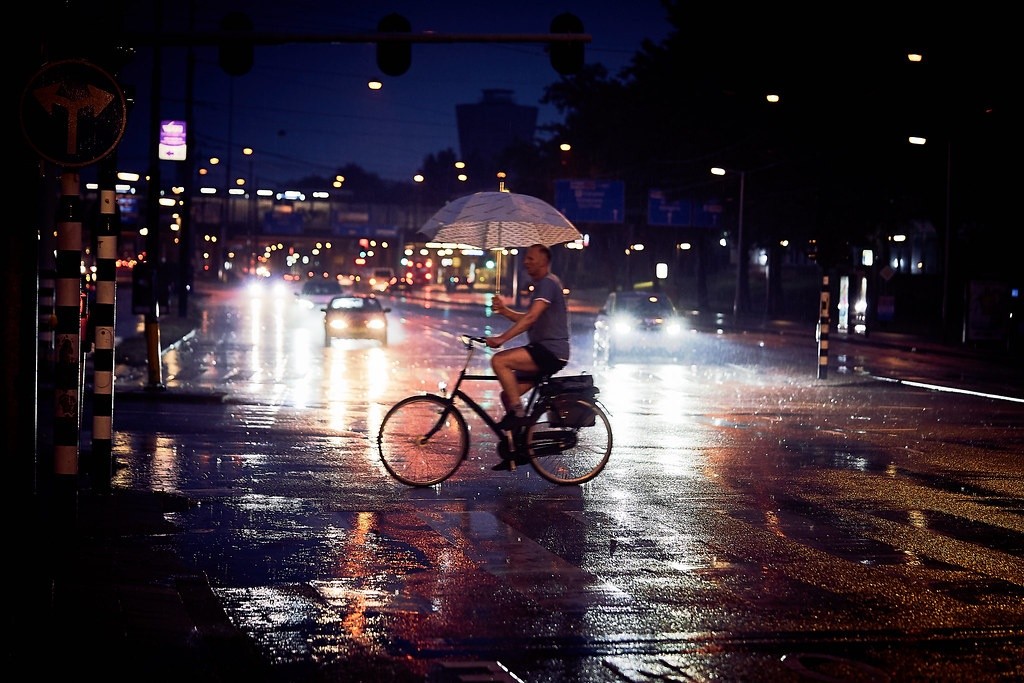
[295,279,347,315]
[321,297,391,347]
[593,290,695,371]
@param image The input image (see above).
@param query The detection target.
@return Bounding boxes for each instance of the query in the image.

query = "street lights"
[709,167,745,321]
[908,136,954,340]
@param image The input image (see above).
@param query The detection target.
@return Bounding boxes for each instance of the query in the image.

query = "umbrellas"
[419,185,584,314]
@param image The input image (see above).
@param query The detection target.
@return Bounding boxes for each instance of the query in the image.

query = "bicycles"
[376,333,614,487]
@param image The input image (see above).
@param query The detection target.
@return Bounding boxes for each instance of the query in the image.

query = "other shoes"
[494,411,533,430]
[491,451,531,471]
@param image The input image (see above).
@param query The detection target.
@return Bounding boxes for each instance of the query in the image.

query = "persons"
[363,299,372,307]
[486,244,569,470]
